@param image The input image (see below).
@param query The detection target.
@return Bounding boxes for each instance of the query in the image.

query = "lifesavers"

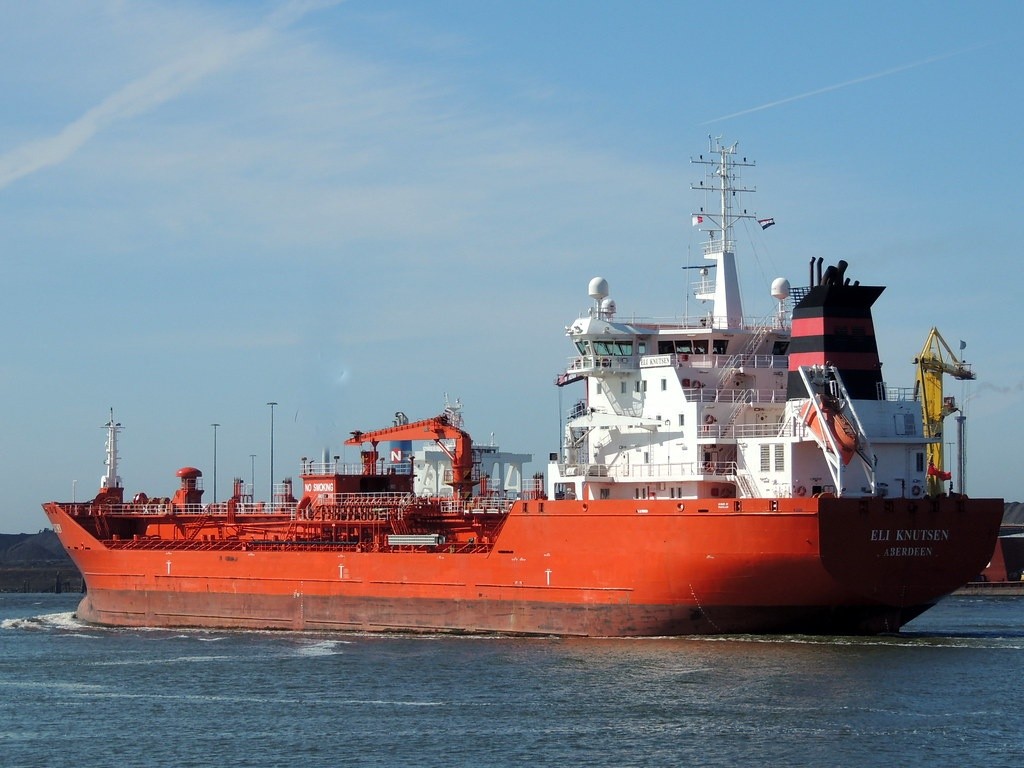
[692,380,701,392]
[706,415,714,424]
[797,485,807,496]
[912,485,921,496]
[721,488,730,498]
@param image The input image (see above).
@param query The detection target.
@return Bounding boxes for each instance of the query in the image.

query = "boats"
[42,134,1005,637]
